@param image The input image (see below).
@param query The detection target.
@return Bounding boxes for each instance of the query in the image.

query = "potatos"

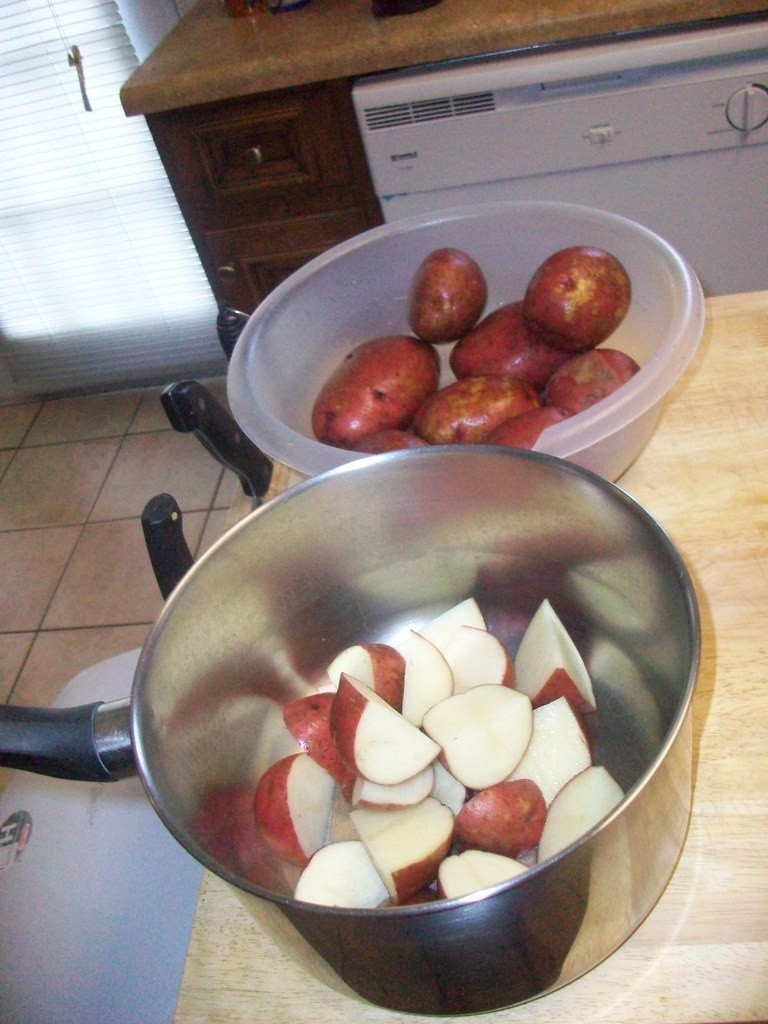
[311,247,641,451]
[256,596,626,910]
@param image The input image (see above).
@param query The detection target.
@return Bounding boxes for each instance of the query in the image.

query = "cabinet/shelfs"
[147,64,384,337]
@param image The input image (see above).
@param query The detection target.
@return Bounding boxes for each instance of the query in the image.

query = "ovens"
[348,17,767,297]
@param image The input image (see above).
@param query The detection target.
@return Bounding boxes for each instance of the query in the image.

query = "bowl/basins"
[225,198,708,484]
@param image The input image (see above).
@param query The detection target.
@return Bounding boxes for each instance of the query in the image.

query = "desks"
[167,291,768,1024]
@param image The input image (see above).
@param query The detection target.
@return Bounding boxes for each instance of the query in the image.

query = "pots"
[0,440,707,1020]
